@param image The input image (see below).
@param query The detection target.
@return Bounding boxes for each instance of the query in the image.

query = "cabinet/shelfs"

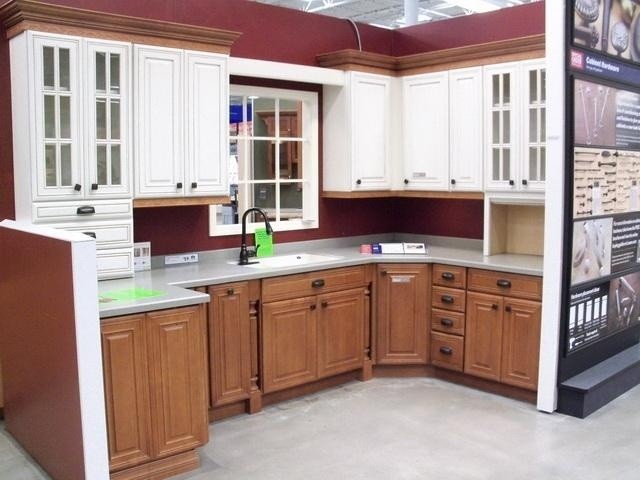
[484,55,546,194]
[260,265,367,390]
[352,67,483,192]
[202,281,254,407]
[377,263,428,365]
[135,43,228,200]
[431,264,465,373]
[467,268,543,391]
[25,32,133,281]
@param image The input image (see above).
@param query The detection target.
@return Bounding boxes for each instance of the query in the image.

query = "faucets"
[238,206,274,266]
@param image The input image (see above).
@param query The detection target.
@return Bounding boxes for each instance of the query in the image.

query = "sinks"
[223,253,348,270]
